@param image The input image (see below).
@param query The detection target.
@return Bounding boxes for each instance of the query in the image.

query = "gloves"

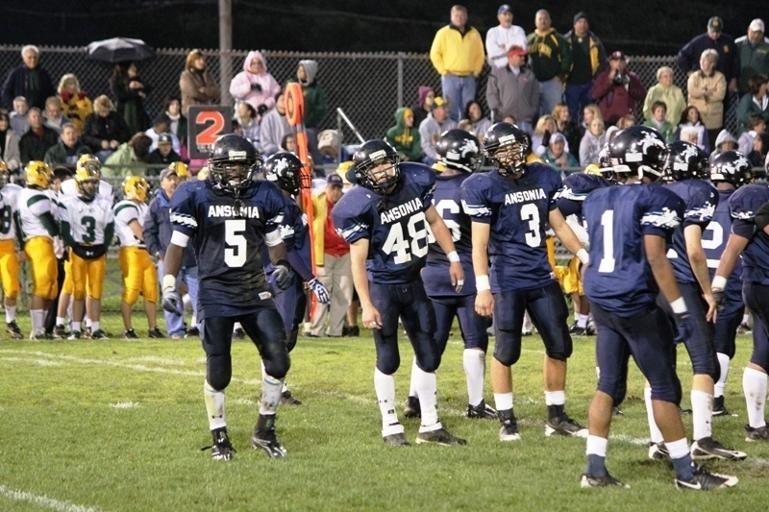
[54,236,64,258]
[162,291,184,317]
[671,311,694,344]
[308,277,331,303]
[269,263,291,290]
[711,291,727,313]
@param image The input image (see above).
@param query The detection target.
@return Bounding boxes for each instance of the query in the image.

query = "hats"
[609,51,624,61]
[498,5,513,13]
[507,48,528,57]
[418,86,432,107]
[750,19,765,33]
[327,174,343,188]
[404,109,414,119]
[158,134,172,145]
[431,97,448,109]
[54,164,73,177]
[707,16,723,33]
[160,169,177,181]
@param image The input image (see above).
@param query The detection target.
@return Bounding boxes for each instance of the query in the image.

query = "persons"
[258,151,331,406]
[460,121,593,440]
[710,152,768,444]
[330,140,468,450]
[642,140,747,463]
[1,42,361,339]
[402,128,499,421]
[546,125,692,418]
[705,148,756,418]
[379,1,768,337]
[579,128,738,493]
[160,133,296,463]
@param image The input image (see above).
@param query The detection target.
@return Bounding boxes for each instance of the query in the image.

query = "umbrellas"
[85,36,158,94]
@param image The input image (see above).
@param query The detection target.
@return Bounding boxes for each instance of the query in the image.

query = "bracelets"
[576,248,589,265]
[162,274,177,291]
[475,275,490,293]
[669,297,688,314]
[711,275,728,292]
[447,251,460,263]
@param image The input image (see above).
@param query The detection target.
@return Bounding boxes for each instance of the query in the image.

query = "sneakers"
[712,396,739,419]
[500,418,521,441]
[648,442,671,461]
[568,320,587,337]
[415,428,467,449]
[579,474,630,493]
[212,439,238,463]
[124,329,139,340]
[745,424,769,442]
[67,330,80,340]
[84,326,110,341]
[586,316,598,335]
[52,325,65,338]
[149,328,165,338]
[251,435,289,462]
[381,422,411,449]
[403,396,421,419]
[677,405,693,419]
[610,407,625,422]
[30,332,62,343]
[736,322,754,336]
[467,399,499,421]
[675,465,738,490]
[547,413,589,439]
[257,391,303,409]
[690,437,747,460]
[6,322,23,339]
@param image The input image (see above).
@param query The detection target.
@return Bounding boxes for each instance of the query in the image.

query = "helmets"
[169,162,192,181]
[209,135,263,199]
[711,151,753,189]
[24,160,54,189]
[263,153,311,196]
[434,129,482,174]
[0,158,9,188]
[77,166,99,193]
[77,154,101,170]
[121,176,152,204]
[353,139,402,197]
[483,123,528,178]
[598,126,669,184]
[662,142,707,181]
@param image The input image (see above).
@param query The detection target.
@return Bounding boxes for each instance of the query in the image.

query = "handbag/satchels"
[71,241,105,260]
